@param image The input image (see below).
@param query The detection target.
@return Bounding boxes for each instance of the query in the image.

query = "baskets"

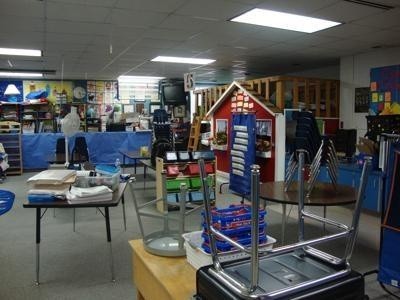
[182,231,277,271]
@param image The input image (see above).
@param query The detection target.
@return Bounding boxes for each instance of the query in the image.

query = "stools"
[128,176,216,257]
[198,152,371,299]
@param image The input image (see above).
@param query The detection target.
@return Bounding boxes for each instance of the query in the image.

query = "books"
[67,184,113,205]
[27,168,77,200]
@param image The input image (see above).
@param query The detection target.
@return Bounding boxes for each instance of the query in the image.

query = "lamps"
[4,84,21,102]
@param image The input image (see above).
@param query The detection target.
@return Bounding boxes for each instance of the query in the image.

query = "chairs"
[48,138,69,164]
[69,137,89,170]
[283,111,342,198]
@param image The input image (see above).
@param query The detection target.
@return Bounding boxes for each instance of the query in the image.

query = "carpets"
[0,190,15,215]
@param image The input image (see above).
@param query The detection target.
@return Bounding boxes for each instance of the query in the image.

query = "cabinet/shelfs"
[0,102,20,123]
[53,103,86,132]
[0,121,23,177]
[155,156,217,214]
[317,166,379,211]
[20,102,53,133]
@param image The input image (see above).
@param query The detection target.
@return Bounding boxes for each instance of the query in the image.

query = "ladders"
[186,115,202,153]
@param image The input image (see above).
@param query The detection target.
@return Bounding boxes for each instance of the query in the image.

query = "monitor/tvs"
[160,80,189,106]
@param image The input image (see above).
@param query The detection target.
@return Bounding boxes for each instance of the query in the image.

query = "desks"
[128,238,198,300]
[119,151,151,175]
[23,173,131,287]
[257,181,359,247]
[139,159,156,191]
